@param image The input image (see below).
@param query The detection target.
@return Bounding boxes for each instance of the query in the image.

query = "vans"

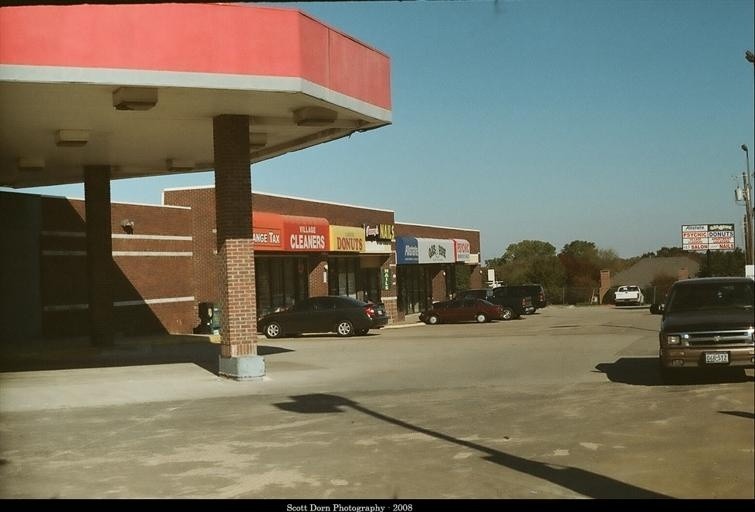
[493,284,545,311]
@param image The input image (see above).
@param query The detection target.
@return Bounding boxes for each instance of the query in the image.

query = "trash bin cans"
[193,302,214,334]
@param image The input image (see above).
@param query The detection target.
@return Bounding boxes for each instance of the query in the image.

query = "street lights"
[741,144,754,266]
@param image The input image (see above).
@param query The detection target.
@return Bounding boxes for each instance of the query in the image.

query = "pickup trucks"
[454,288,546,315]
[613,285,644,306]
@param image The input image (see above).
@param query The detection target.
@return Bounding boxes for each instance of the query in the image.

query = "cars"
[650,276,755,378]
[419,296,504,324]
[257,295,388,339]
